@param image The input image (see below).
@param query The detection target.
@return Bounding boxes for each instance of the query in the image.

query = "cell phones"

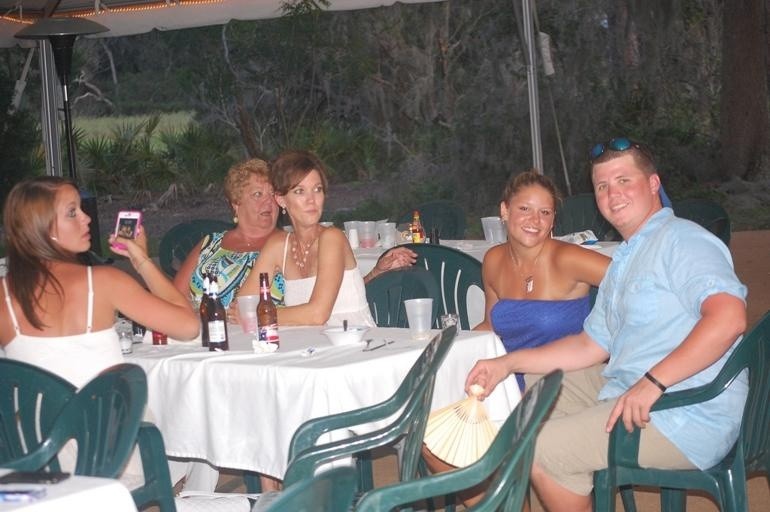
[111,207,142,250]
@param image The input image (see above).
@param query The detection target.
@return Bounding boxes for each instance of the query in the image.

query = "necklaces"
[292,238,310,268]
[512,247,542,295]
[241,234,263,247]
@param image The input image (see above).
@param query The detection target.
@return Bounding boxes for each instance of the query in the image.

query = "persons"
[172,158,420,325]
[0,174,200,474]
[420,170,612,512]
[464,140,749,512]
[225,150,378,493]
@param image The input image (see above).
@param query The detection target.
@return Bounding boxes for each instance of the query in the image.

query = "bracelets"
[137,258,152,274]
[645,372,667,391]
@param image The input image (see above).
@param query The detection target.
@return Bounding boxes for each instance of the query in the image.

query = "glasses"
[589,136,651,164]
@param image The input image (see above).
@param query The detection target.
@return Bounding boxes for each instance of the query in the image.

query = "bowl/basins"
[319,326,371,347]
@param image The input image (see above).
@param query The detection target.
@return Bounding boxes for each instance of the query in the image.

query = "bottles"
[430,223,441,245]
[118,311,146,343]
[151,330,167,345]
[408,212,426,248]
[200,274,231,353]
[256,272,280,350]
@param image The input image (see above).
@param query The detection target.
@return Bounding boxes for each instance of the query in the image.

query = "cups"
[113,321,133,355]
[480,216,508,246]
[342,218,415,251]
[404,298,433,339]
[319,221,334,228]
[283,225,294,233]
[439,314,458,336]
[236,295,261,335]
